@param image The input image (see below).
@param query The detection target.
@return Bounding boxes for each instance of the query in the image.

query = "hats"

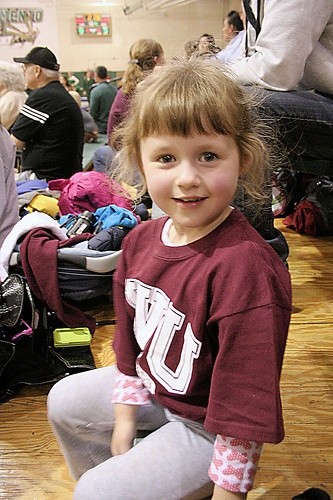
[13,47,60,71]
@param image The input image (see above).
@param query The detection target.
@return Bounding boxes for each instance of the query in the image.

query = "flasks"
[66,211,98,240]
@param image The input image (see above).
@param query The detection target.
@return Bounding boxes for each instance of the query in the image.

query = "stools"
[282,166,333,218]
[134,429,158,448]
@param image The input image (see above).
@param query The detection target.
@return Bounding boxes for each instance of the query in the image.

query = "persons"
[89,66,118,133]
[217,0,333,270]
[1,59,94,129]
[10,47,84,181]
[47,50,293,500]
[186,10,248,64]
[107,39,164,152]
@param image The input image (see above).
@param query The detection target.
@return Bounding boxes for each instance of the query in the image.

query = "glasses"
[20,65,34,73]
